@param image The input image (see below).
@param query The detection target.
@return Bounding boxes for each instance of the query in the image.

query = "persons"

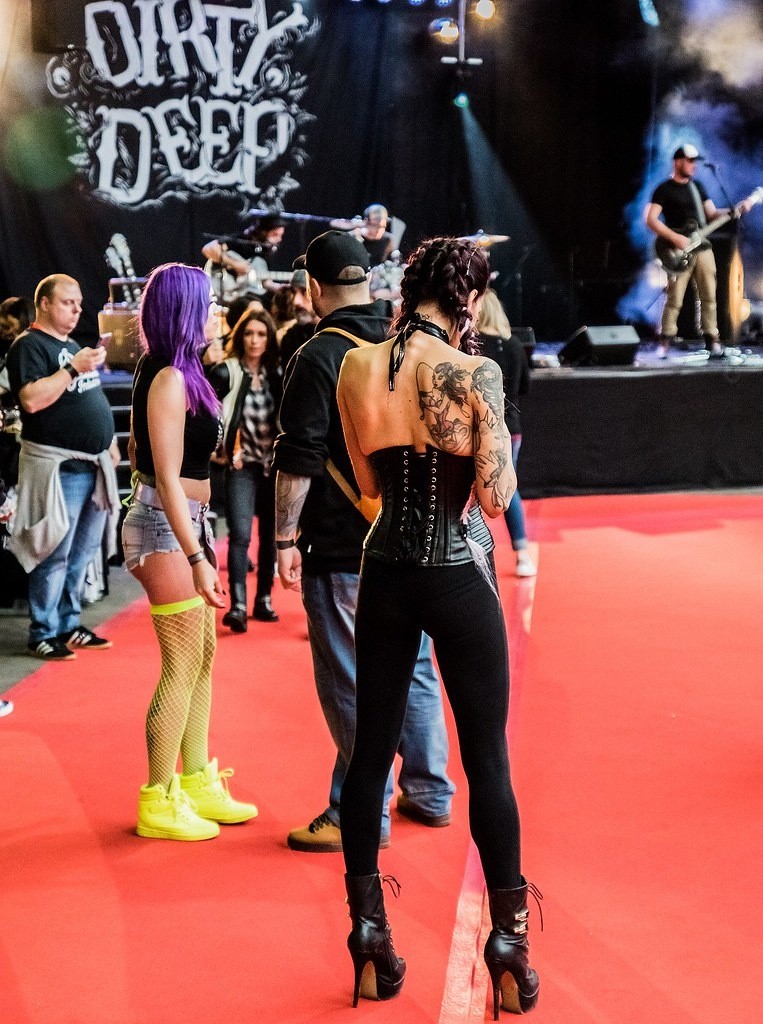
[333,236,542,1024]
[201,210,292,292]
[286,231,460,854]
[348,204,402,302]
[119,260,258,843]
[208,307,287,633]
[4,272,124,661]
[218,291,270,352]
[0,294,35,506]
[459,286,539,579]
[278,254,323,377]
[645,144,753,361]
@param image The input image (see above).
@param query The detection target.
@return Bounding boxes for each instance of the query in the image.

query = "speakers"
[557,324,641,368]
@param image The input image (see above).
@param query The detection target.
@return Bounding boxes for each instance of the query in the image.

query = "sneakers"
[176,757,259,824]
[26,633,79,663]
[512,549,536,578]
[286,806,392,857]
[396,791,452,829]
[58,627,113,651]
[136,776,221,843]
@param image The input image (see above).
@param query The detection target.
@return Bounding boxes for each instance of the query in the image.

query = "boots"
[481,876,540,1023]
[655,330,672,357]
[221,581,250,634]
[252,583,279,621]
[343,867,407,1010]
[702,333,725,357]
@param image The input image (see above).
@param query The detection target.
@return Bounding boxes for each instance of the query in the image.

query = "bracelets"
[62,363,79,379]
[273,538,295,550]
[186,549,207,566]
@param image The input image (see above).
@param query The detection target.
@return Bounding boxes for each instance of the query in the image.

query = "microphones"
[264,243,276,252]
[703,161,720,173]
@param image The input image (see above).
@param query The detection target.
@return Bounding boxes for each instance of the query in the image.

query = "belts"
[130,479,212,528]
[55,460,98,474]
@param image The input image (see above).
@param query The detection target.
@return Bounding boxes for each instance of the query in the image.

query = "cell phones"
[94,333,112,349]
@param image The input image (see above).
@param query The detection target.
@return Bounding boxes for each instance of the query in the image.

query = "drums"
[367,263,404,304]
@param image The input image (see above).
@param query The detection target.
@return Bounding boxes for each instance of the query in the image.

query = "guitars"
[202,251,297,306]
[652,185,762,274]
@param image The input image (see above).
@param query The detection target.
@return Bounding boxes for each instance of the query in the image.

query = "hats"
[301,229,374,286]
[673,143,704,163]
[254,209,286,230]
[364,205,389,228]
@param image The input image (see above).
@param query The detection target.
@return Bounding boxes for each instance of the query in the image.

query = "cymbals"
[330,219,381,233]
[460,233,509,248]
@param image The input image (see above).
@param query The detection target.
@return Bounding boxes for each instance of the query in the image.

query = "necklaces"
[397,315,450,345]
